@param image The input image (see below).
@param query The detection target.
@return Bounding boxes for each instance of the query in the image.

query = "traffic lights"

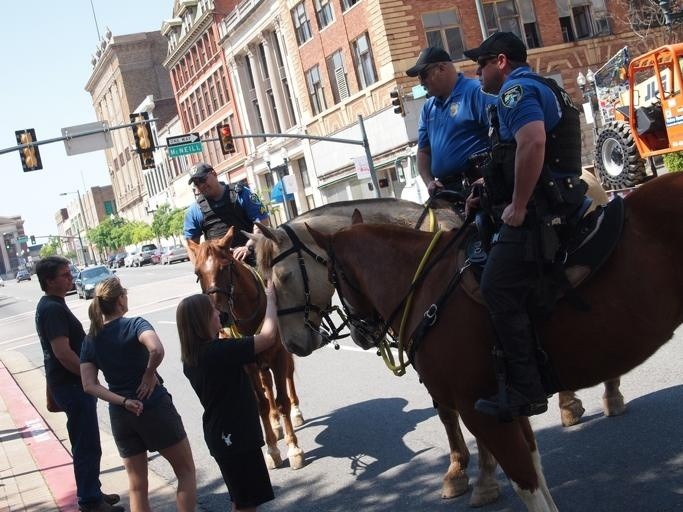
[217,124,236,155]
[5,240,11,249]
[389,91,408,117]
[129,112,156,152]
[139,151,155,170]
[15,128,43,172]
[30,235,35,245]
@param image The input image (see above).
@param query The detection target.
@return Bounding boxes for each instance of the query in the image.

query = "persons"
[35,256,126,512]
[181,162,271,266]
[176,278,278,512]
[79,277,195,512]
[462,30,582,415]
[405,46,498,197]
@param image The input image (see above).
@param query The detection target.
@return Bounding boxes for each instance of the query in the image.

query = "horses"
[185,225,307,470]
[240,166,627,507]
[304,170,683,512]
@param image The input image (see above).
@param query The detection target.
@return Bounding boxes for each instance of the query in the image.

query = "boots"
[474,309,547,419]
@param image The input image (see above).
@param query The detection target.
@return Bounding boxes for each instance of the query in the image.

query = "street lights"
[111,211,124,226]
[577,69,599,138]
[60,189,98,266]
[263,147,292,221]
[145,200,161,245]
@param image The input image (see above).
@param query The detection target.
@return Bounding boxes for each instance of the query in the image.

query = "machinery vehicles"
[592,41,683,190]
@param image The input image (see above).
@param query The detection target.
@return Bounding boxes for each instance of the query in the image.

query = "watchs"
[246,244,256,252]
[122,397,127,406]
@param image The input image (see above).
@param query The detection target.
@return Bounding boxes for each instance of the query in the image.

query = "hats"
[189,164,213,186]
[460,30,529,64]
[406,46,450,79]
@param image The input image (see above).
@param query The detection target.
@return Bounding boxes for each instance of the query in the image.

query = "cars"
[70,265,81,291]
[113,252,128,268]
[124,251,137,267]
[16,270,31,283]
[75,266,116,299]
[159,244,189,264]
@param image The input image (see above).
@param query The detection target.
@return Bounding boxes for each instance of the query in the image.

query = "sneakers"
[101,492,121,505]
[79,502,125,512]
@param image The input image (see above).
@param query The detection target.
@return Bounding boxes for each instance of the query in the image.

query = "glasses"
[194,171,215,188]
[417,66,439,82]
[476,55,499,70]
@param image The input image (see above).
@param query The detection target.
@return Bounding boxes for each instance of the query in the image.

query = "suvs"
[133,243,157,267]
